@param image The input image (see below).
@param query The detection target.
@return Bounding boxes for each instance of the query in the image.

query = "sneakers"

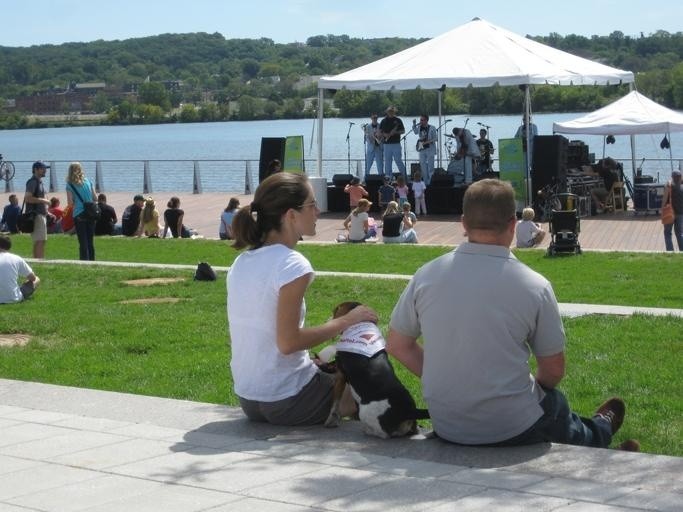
[593,396,625,436]
[595,207,605,213]
[612,440,641,453]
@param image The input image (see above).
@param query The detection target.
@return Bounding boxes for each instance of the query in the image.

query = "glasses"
[298,199,318,209]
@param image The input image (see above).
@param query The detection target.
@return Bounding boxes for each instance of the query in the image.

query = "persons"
[516,207,546,249]
[121,194,145,235]
[17,161,57,258]
[218,197,241,240]
[345,178,368,211]
[412,115,437,185]
[0,234,39,306]
[382,202,419,245]
[62,162,102,260]
[343,199,377,243]
[380,106,407,178]
[401,202,416,231]
[158,196,199,240]
[1,194,22,234]
[453,127,478,184]
[663,169,683,252]
[227,172,378,427]
[95,194,122,235]
[364,114,384,176]
[386,178,642,451]
[379,174,395,209]
[47,197,64,234]
[411,171,429,216]
[392,177,409,207]
[138,198,162,238]
[515,115,538,169]
[475,129,495,172]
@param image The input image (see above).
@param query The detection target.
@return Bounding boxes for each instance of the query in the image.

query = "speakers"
[258,136,285,186]
[531,134,568,221]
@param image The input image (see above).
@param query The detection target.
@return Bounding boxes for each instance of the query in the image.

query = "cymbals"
[443,132,456,139]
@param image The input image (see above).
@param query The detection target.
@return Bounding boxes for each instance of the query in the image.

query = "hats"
[671,169,681,177]
[32,162,51,170]
[134,195,147,202]
[383,176,391,182]
[357,198,373,206]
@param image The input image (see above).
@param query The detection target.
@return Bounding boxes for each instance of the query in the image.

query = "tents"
[317,19,639,218]
[551,92,683,206]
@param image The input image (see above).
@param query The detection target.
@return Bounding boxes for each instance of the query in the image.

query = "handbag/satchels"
[660,180,675,225]
[17,178,40,232]
[221,215,234,239]
[67,180,99,215]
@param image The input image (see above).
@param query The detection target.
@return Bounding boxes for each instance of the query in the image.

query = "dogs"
[316,301,431,441]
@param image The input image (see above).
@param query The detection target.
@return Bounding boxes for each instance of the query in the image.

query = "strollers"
[547,193,581,255]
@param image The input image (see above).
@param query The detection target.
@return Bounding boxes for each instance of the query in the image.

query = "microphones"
[476,120,483,127]
[347,121,355,126]
[464,118,470,126]
[362,122,368,129]
[443,118,452,124]
[413,121,422,128]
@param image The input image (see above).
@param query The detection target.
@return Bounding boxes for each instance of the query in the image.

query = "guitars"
[415,134,438,152]
